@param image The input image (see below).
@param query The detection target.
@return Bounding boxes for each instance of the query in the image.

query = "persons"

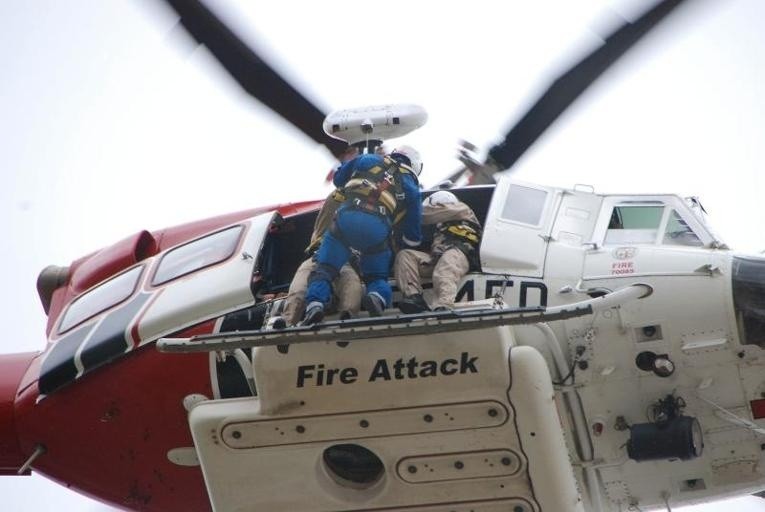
[273,144,481,353]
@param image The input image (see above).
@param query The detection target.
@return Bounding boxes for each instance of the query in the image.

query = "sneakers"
[399,294,454,318]
[362,294,383,317]
[274,308,356,354]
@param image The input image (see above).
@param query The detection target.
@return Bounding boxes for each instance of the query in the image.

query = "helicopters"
[0,0,765,512]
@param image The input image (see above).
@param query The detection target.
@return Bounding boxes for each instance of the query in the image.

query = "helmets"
[422,190,458,209]
[391,146,424,177]
